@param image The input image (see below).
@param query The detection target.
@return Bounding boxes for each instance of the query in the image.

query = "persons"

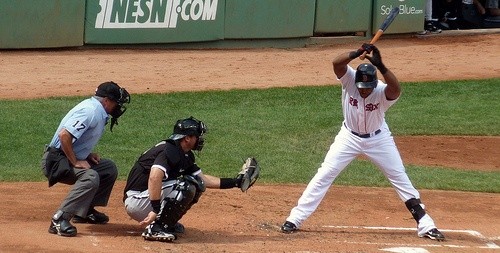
[41,82,131,236]
[419,0,500,35]
[281,42,445,241]
[123,118,259,242]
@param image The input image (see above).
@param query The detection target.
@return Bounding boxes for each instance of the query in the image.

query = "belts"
[343,122,382,138]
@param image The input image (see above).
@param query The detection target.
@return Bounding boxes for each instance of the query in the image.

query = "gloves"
[349,42,387,74]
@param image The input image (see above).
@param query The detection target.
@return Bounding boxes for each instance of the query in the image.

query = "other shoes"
[280,221,297,234]
[48,220,77,237]
[424,18,442,34]
[173,222,184,233]
[416,27,427,35]
[425,228,445,240]
[72,209,110,224]
[142,221,176,243]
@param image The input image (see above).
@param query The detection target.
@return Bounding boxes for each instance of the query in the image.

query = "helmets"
[171,116,206,140]
[355,63,378,88]
[96,81,122,101]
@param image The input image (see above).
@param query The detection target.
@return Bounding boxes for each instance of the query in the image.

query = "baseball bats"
[359,6,401,59]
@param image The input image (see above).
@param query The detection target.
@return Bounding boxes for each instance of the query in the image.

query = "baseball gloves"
[236,158,260,192]
